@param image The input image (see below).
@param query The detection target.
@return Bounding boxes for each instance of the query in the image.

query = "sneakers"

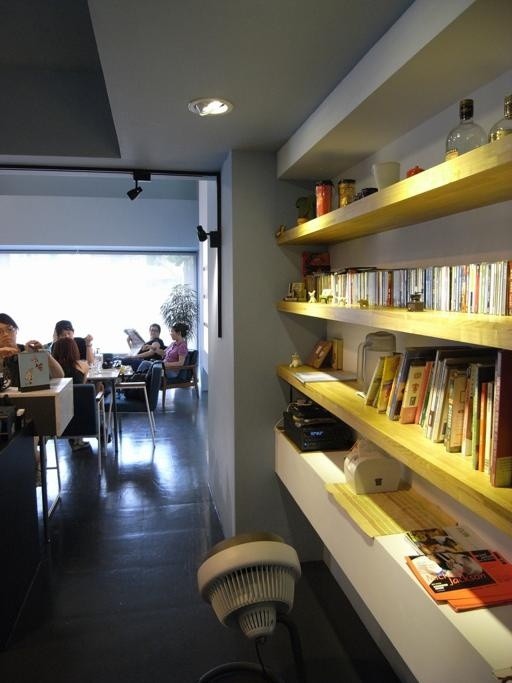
[69,437,90,451]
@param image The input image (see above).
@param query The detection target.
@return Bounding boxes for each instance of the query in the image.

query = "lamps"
[126,169,152,201]
[197,225,219,249]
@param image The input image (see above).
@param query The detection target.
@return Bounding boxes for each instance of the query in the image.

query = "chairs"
[37,348,200,479]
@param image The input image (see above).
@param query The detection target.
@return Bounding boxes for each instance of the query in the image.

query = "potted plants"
[295,195,312,226]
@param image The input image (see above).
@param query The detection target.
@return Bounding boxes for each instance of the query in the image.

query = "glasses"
[0,326,17,337]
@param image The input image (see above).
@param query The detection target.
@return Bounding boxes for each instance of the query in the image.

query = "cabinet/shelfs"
[269,127,511,682]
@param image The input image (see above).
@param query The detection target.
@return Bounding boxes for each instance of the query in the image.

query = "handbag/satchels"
[124,372,151,403]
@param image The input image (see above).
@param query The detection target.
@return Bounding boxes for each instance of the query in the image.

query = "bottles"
[91,347,121,373]
[490,95,512,142]
[356,331,397,395]
[445,97,488,160]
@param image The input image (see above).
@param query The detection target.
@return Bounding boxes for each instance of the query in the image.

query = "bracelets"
[86,344,92,347]
[154,347,157,351]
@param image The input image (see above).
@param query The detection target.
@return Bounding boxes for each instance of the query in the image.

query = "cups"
[315,178,356,218]
[373,161,401,191]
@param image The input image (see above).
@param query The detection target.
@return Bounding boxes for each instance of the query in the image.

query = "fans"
[191,526,307,683]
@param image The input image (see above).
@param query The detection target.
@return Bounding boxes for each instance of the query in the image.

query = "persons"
[136,322,189,379]
[0,313,65,464]
[50,337,111,452]
[110,325,164,371]
[50,320,94,365]
